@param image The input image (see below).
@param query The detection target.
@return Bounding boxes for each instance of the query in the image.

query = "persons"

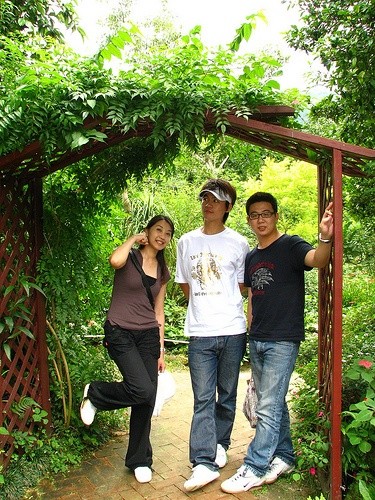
[173,178,251,492]
[80,214,175,484]
[220,191,333,494]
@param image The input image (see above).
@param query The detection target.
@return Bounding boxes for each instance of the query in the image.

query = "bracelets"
[160,348,165,351]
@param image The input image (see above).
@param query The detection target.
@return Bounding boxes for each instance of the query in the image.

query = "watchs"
[319,233,330,243]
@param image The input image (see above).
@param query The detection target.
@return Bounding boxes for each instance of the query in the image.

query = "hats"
[199,186,232,204]
[151,370,177,417]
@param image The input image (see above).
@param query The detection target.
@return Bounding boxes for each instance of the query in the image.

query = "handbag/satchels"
[242,371,258,428]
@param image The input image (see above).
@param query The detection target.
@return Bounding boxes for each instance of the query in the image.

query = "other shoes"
[80,384,97,425]
[134,466,153,483]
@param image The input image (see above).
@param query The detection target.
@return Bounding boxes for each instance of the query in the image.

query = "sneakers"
[183,464,220,492]
[220,465,268,495]
[215,443,229,467]
[264,456,296,484]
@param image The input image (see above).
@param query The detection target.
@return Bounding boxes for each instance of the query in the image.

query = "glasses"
[249,212,275,220]
